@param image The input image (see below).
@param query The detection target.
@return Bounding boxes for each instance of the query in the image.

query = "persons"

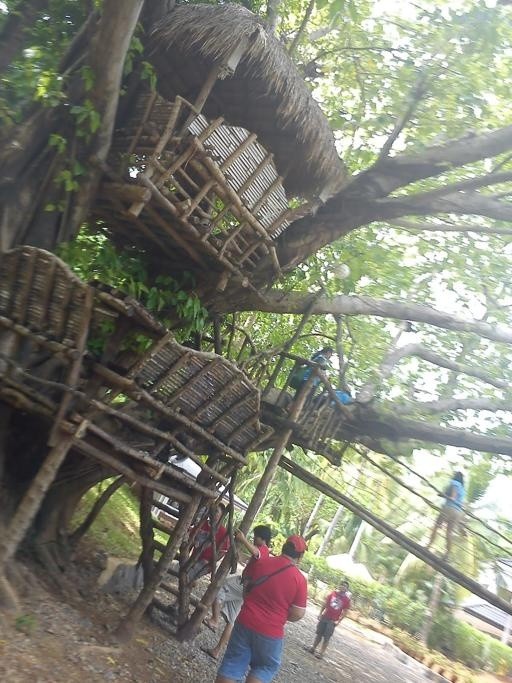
[184,504,307,683]
[426,472,465,562]
[285,347,353,413]
[302,581,350,658]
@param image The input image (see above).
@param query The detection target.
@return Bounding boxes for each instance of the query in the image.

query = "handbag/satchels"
[288,367,304,389]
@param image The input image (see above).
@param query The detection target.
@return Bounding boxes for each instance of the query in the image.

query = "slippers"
[168,604,175,608]
[202,619,216,633]
[200,645,217,659]
[315,653,323,659]
[304,646,315,654]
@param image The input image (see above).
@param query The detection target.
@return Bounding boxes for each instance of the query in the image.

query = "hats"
[286,535,307,553]
